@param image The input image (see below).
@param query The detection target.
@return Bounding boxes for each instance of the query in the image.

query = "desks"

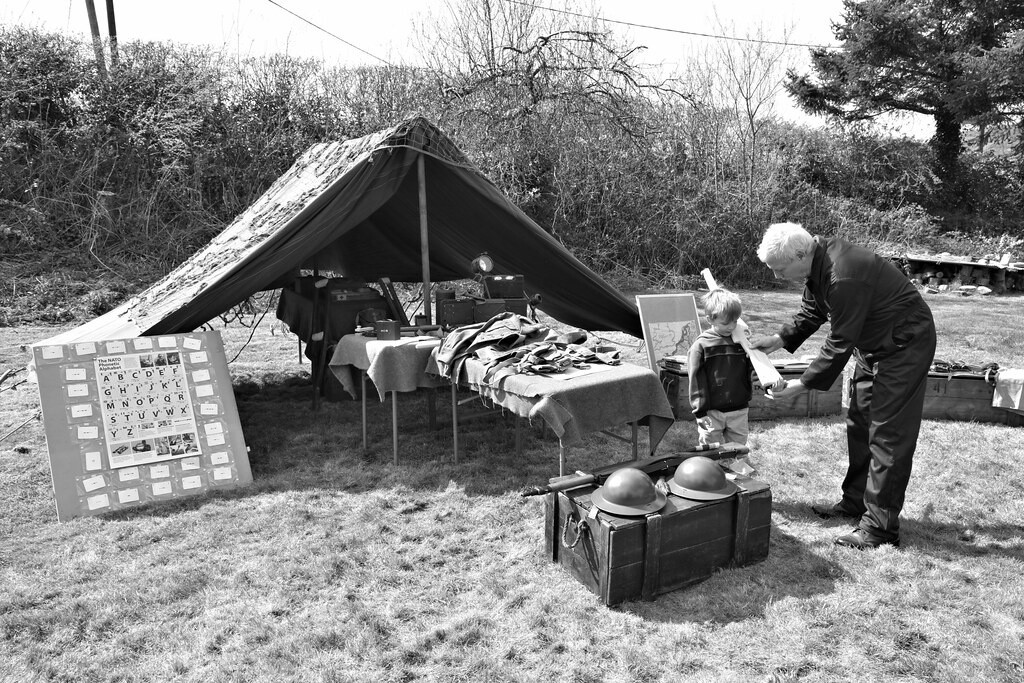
[283,275,389,395]
[339,325,654,477]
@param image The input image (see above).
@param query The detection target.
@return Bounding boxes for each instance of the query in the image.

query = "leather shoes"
[812,504,862,522]
[837,527,900,551]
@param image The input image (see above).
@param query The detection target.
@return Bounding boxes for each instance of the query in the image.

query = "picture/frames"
[635,292,702,371]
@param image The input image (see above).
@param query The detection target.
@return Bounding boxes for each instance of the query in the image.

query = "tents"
[38,110,647,347]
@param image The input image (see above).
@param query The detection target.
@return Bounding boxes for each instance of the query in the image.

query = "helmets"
[666,456,738,500]
[591,467,667,515]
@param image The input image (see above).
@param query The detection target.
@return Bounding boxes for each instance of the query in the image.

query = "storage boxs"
[544,449,772,608]
[484,275,524,299]
[659,370,696,423]
[439,300,528,326]
[748,355,844,420]
[921,360,1024,427]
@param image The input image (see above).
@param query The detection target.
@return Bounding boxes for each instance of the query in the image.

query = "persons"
[745,217,936,551]
[685,287,787,445]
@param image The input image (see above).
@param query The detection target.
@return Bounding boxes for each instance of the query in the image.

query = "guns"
[699,267,786,389]
[519,440,750,498]
